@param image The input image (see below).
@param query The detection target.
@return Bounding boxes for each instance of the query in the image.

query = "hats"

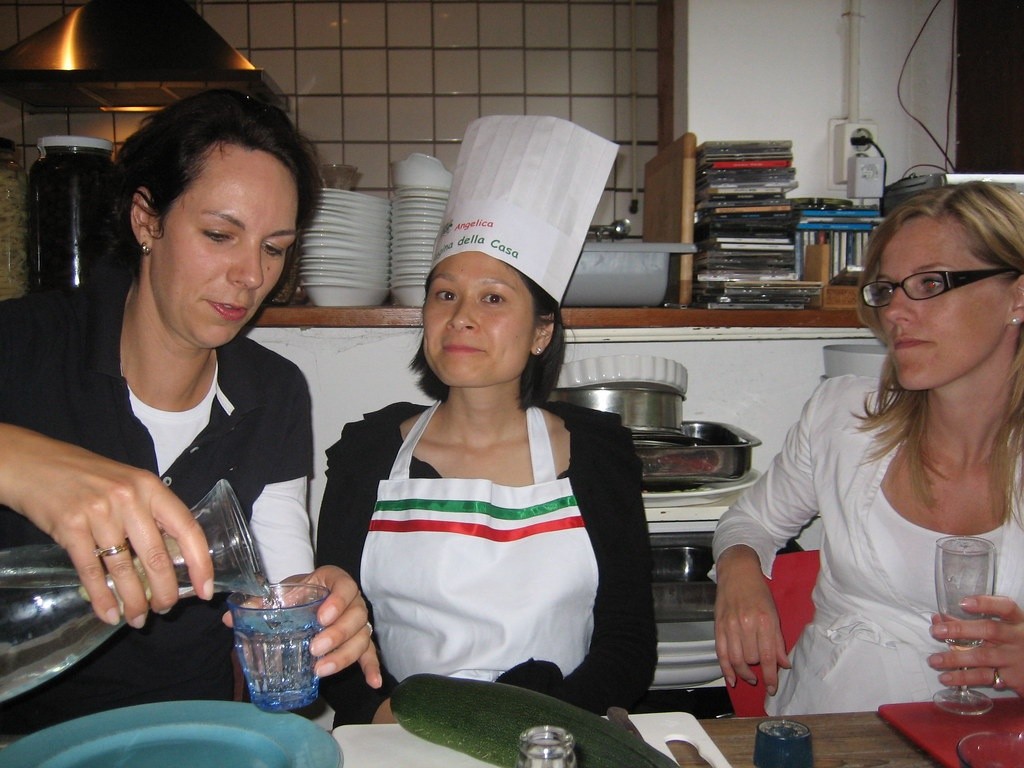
[428,114,620,310]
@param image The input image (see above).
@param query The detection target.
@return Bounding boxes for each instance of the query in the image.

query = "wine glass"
[931,536,994,715]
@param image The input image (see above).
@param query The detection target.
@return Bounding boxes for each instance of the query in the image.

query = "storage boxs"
[561,243,697,307]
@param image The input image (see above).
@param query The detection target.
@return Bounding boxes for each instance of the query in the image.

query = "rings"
[93,542,129,557]
[366,621,373,636]
[992,668,1007,689]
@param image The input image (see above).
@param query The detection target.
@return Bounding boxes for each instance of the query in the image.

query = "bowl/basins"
[298,190,391,308]
[386,153,453,307]
[649,545,720,622]
[550,386,686,434]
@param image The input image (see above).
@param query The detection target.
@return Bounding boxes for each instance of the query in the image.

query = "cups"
[227,582,331,711]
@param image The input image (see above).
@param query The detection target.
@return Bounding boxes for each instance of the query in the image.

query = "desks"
[667,711,946,768]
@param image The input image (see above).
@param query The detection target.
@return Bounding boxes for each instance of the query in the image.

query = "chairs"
[721,550,820,718]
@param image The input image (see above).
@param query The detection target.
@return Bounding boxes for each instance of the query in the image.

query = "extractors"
[0,0,289,115]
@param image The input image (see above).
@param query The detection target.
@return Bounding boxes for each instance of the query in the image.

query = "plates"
[0,700,344,768]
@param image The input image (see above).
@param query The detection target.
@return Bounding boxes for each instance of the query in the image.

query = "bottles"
[0,136,117,300]
[320,163,359,191]
[0,477,274,706]
[956,731,1024,768]
[514,724,578,768]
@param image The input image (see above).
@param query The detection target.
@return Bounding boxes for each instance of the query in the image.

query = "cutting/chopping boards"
[332,712,735,768]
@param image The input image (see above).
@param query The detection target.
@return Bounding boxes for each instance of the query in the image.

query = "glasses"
[860,266,1016,308]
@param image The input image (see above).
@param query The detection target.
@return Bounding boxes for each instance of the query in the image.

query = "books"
[697,140,886,310]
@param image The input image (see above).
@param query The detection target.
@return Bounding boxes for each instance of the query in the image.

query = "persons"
[316,251,659,724]
[707,181,1024,718]
[0,89,382,734]
